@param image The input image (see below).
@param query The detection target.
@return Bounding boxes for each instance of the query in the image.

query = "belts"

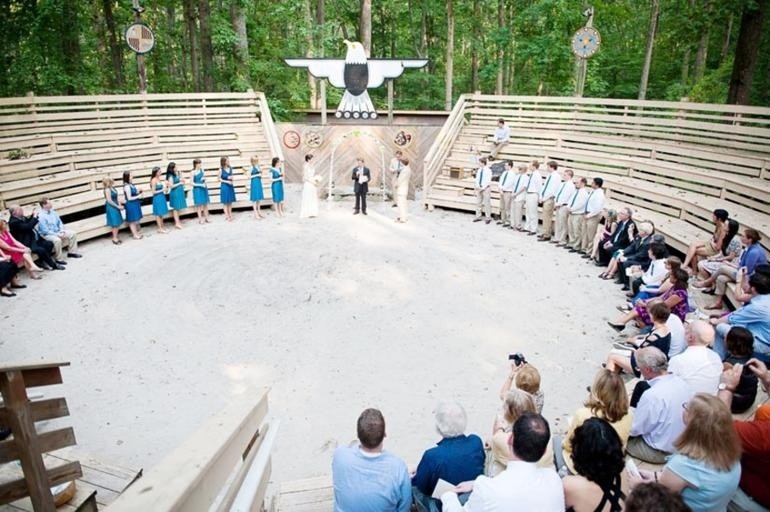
[527,192,537,195]
[562,203,568,206]
[501,189,512,194]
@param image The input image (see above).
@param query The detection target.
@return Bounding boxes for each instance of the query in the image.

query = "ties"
[651,264,654,277]
[555,183,566,204]
[501,171,509,188]
[584,190,593,214]
[541,175,552,199]
[478,168,483,188]
[396,160,401,179]
[513,174,521,193]
[570,190,579,208]
[525,173,533,192]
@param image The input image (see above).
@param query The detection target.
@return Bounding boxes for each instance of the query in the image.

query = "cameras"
[509,353,527,367]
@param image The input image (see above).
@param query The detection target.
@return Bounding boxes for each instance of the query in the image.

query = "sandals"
[229,215,236,220]
[254,214,261,220]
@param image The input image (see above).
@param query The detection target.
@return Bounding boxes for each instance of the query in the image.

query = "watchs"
[717,381,732,393]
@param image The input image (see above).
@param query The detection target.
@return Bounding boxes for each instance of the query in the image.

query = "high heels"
[201,220,205,225]
[256,216,262,220]
[615,304,632,313]
[112,239,122,247]
[607,320,625,332]
[174,224,182,231]
[164,228,169,232]
[204,217,211,222]
[158,228,165,235]
[259,213,266,219]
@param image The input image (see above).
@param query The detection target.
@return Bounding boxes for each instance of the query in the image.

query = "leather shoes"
[1,290,17,297]
[564,246,573,250]
[703,304,724,309]
[485,219,490,225]
[626,299,631,302]
[595,263,607,267]
[549,240,559,244]
[577,251,586,255]
[519,229,528,233]
[132,234,143,240]
[537,234,543,238]
[66,252,84,261]
[496,221,503,225]
[10,284,28,290]
[527,232,536,236]
[507,226,512,229]
[29,274,44,281]
[353,211,360,215]
[621,287,629,291]
[472,218,481,223]
[692,281,713,288]
[56,259,67,265]
[487,155,495,162]
[599,272,604,278]
[502,223,510,226]
[614,280,623,284]
[513,227,520,231]
[555,244,566,248]
[362,211,368,216]
[537,237,551,241]
[701,287,716,295]
[602,273,614,280]
[568,249,580,253]
[390,203,398,208]
[28,267,45,272]
[52,264,63,272]
[581,254,590,259]
[626,292,633,297]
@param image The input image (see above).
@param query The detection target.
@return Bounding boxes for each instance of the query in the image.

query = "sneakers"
[589,258,593,261]
[613,342,638,353]
[688,274,699,283]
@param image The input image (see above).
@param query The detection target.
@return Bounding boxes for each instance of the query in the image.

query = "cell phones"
[738,363,754,372]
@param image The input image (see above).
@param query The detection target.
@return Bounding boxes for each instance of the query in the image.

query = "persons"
[166,161,187,230]
[499,360,545,415]
[601,301,670,378]
[598,205,638,266]
[497,160,514,227]
[579,177,607,259]
[681,208,728,279]
[510,164,529,230]
[395,157,410,223]
[563,177,588,253]
[698,220,742,296]
[624,346,693,464]
[537,161,560,241]
[102,177,124,245]
[488,118,510,161]
[8,204,66,271]
[150,167,169,234]
[268,157,287,217]
[248,155,264,220]
[616,254,681,311]
[520,160,542,236]
[642,392,741,512]
[332,408,412,512]
[550,169,575,247]
[645,298,687,360]
[411,402,486,512]
[715,326,759,414]
[556,416,630,512]
[298,155,322,218]
[711,272,770,355]
[36,195,82,265]
[616,223,653,286]
[352,157,371,215]
[123,169,145,240]
[589,207,618,261]
[485,391,553,469]
[718,359,770,511]
[627,232,665,296]
[608,269,688,332]
[390,151,403,207]
[473,157,493,225]
[441,413,565,512]
[0,218,43,280]
[631,242,667,301]
[551,369,635,474]
[735,265,770,308]
[600,221,655,279]
[190,159,213,223]
[218,156,236,222]
[670,319,723,399]
[0,247,27,297]
[690,229,769,309]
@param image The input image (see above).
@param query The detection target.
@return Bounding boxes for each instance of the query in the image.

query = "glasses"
[681,402,690,414]
[518,168,522,171]
[634,367,642,373]
[662,261,669,265]
